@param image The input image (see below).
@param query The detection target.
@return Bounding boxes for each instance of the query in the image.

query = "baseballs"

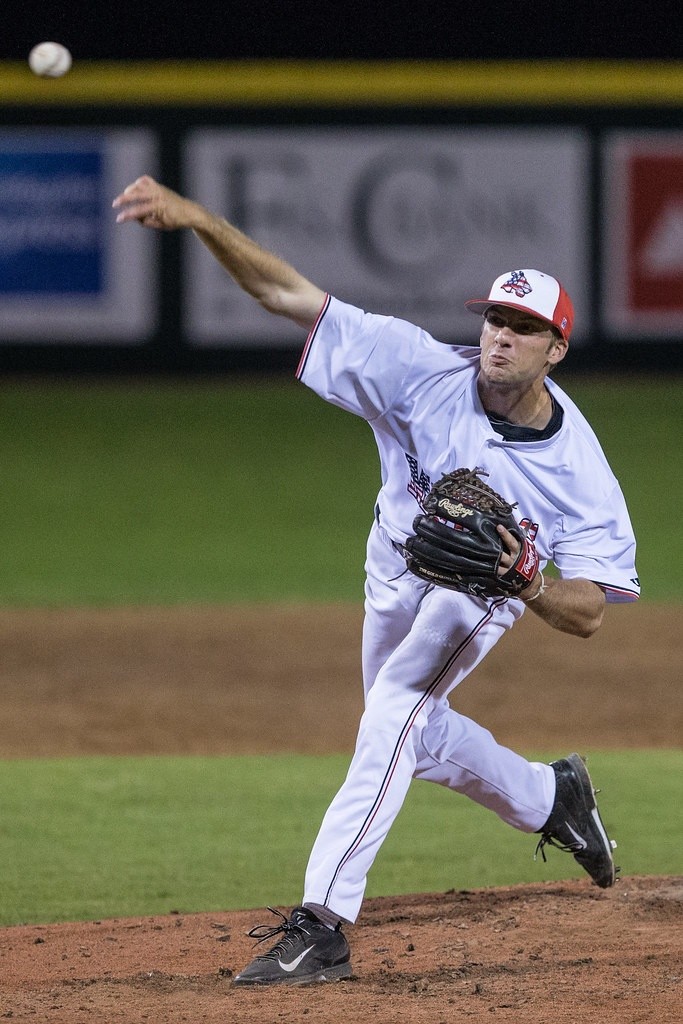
[28,41,72,79]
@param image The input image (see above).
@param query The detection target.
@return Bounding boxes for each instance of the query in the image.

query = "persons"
[112,175,642,987]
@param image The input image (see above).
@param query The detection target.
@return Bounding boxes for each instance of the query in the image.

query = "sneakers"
[229,905,353,988]
[534,751,621,889]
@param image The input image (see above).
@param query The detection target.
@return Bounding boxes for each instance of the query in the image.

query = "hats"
[463,269,574,341]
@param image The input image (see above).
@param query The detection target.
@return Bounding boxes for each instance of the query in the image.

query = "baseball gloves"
[387,465,540,603]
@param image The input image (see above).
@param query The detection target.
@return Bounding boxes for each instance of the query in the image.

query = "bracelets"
[524,570,548,601]
